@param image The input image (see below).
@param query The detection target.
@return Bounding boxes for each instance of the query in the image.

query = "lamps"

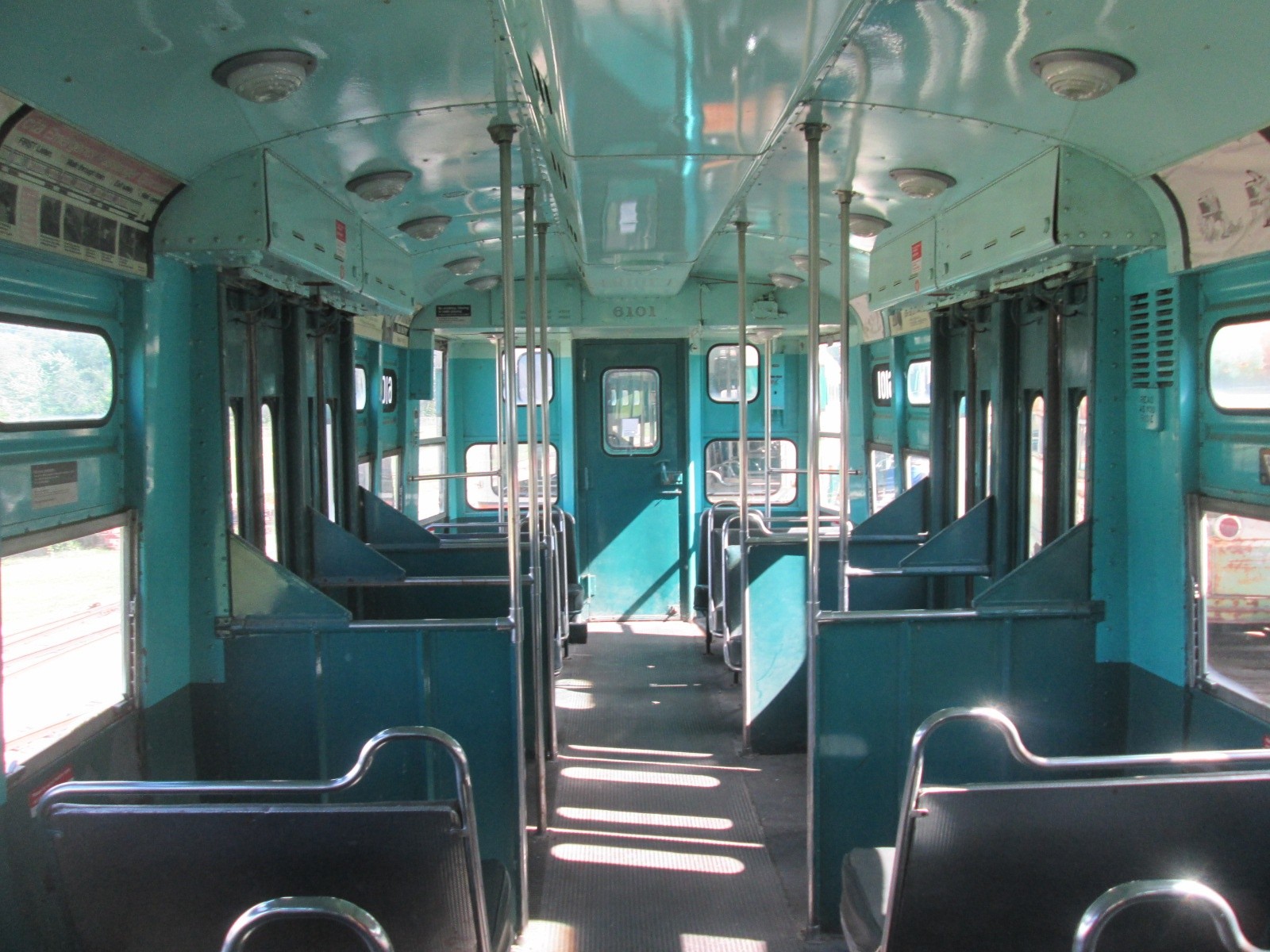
[211,49,318,104]
[345,171,413,204]
[889,168,956,199]
[464,275,502,292]
[768,273,804,289]
[443,256,485,276]
[398,216,453,241]
[1029,48,1136,101]
[838,213,892,237]
[788,255,832,272]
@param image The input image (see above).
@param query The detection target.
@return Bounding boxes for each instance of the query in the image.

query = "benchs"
[694,498,1270,952]
[24,510,591,952]
